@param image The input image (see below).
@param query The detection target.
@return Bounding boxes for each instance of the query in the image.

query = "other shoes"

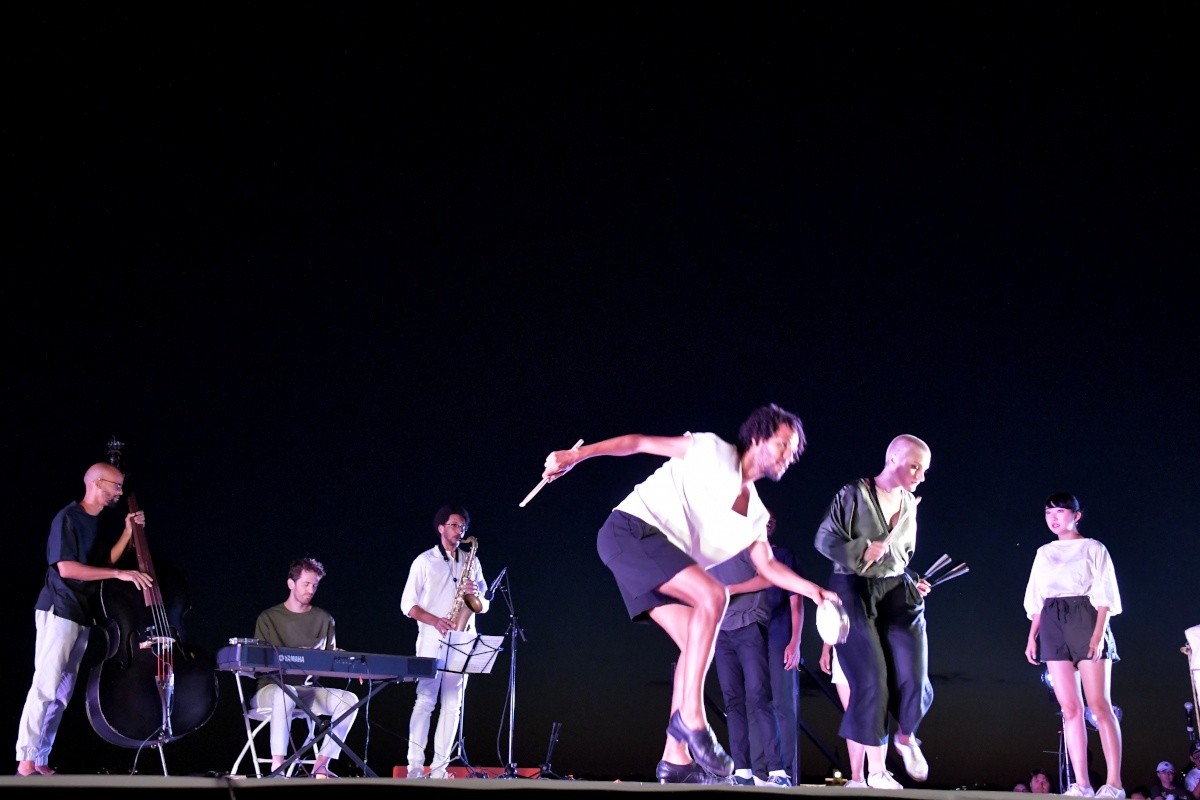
[34,763,57,776]
[429,769,449,780]
[15,771,41,776]
[271,772,285,778]
[766,775,793,787]
[723,775,755,786]
[310,772,338,779]
[842,779,867,789]
[407,763,424,780]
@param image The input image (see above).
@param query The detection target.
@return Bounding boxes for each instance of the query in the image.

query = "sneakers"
[894,733,929,782]
[1094,784,1126,799]
[1063,783,1095,798]
[867,771,904,790]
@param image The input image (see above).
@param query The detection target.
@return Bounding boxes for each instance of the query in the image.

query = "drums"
[815,599,851,646]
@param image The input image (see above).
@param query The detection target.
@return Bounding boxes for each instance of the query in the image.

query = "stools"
[231,707,320,775]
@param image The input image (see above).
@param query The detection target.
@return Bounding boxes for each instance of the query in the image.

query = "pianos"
[215,643,439,778]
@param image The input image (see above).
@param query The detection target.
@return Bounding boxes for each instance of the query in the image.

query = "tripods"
[416,632,503,778]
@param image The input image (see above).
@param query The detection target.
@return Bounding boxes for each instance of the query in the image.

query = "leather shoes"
[656,759,731,786]
[666,708,734,780]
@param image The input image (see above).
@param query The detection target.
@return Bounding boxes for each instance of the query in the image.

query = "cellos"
[86,438,218,778]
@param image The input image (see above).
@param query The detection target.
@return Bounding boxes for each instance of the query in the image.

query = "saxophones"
[446,536,483,631]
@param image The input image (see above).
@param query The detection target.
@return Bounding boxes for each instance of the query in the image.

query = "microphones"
[484,566,507,600]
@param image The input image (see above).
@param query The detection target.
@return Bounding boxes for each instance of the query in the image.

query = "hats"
[1156,761,1175,773]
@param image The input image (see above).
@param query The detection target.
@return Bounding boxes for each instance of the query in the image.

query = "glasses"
[440,522,469,531]
[97,478,123,489]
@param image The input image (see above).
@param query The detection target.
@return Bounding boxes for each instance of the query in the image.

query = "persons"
[250,558,363,779]
[1023,490,1126,800]
[399,505,489,780]
[542,404,934,789]
[16,463,153,778]
[1012,745,1200,800]
